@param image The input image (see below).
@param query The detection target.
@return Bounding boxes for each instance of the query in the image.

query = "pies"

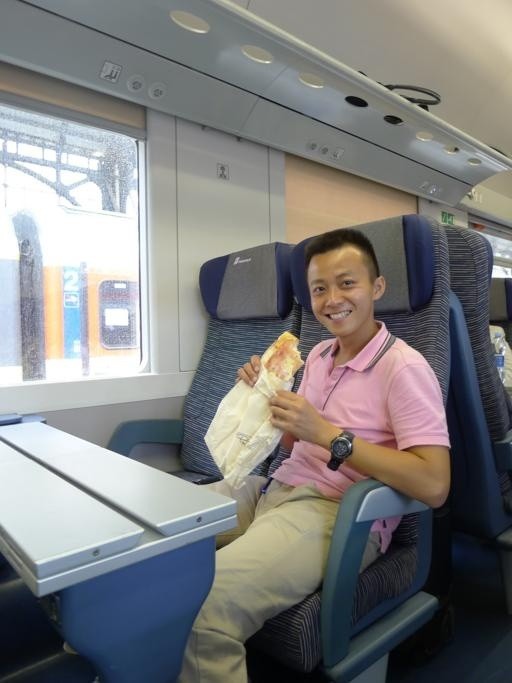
[265,333,305,381]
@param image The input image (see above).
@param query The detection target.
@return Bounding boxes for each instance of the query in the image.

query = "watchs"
[325,428,356,472]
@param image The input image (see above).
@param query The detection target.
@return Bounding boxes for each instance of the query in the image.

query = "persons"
[58,225,453,682]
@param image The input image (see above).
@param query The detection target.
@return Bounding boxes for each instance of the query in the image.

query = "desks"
[0,414,240,683]
